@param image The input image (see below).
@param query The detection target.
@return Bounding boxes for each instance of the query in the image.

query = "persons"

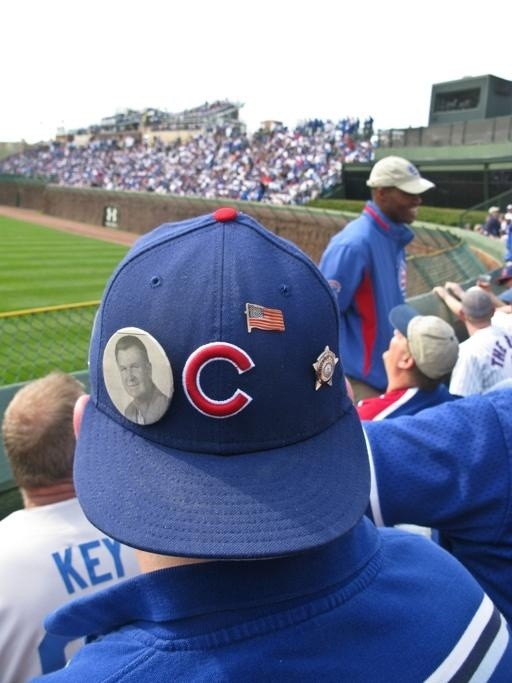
[0,368,144,683]
[360,376,512,622]
[115,335,169,423]
[356,199,512,419]
[1,99,377,205]
[314,152,434,398]
[25,207,512,683]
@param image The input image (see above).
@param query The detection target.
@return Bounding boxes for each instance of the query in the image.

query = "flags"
[246,302,288,333]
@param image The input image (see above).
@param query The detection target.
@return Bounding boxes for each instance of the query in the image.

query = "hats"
[366,156,436,195]
[497,288,512,304]
[488,204,512,221]
[73,208,372,559]
[495,264,512,286]
[462,286,493,316]
[388,303,459,379]
